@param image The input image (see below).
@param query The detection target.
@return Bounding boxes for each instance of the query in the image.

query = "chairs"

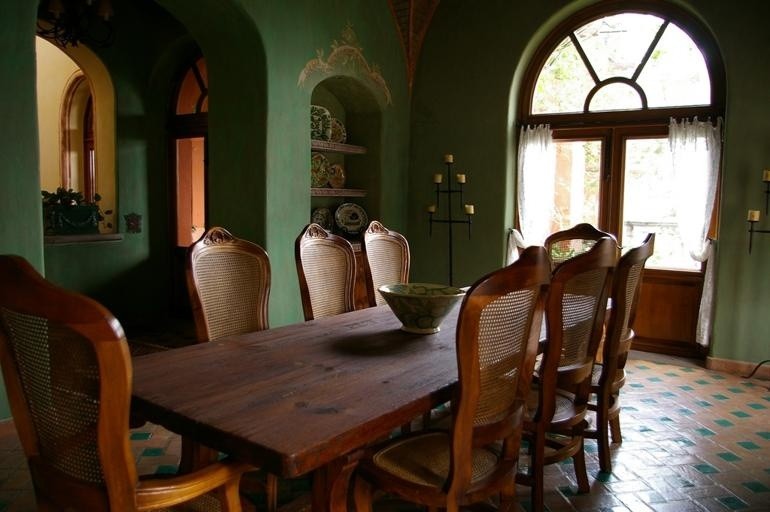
[1,252,263,510]
[186,226,272,340]
[293,224,358,319]
[453,236,625,510]
[526,233,657,480]
[362,222,413,305]
[544,221,622,294]
[368,246,553,508]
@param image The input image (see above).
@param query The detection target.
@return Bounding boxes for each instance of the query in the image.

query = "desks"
[96,287,616,511]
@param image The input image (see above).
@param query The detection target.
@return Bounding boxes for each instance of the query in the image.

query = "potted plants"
[41,187,113,234]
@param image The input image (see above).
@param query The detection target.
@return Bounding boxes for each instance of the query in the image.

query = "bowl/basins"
[377,281,466,335]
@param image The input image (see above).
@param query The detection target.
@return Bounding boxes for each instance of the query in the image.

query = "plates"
[310,105,332,141]
[334,203,369,234]
[312,207,334,234]
[331,117,347,144]
[327,163,346,189]
[311,151,331,188]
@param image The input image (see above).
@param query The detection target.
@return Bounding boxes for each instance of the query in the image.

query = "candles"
[747,210,760,221]
[763,169,770,181]
[465,205,474,214]
[457,174,465,183]
[444,155,453,163]
[435,174,442,183]
[428,206,435,211]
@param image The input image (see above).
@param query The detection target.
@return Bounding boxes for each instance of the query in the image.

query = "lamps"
[37,1,115,52]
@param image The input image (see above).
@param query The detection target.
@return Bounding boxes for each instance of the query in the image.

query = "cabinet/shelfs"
[311,138,370,196]
